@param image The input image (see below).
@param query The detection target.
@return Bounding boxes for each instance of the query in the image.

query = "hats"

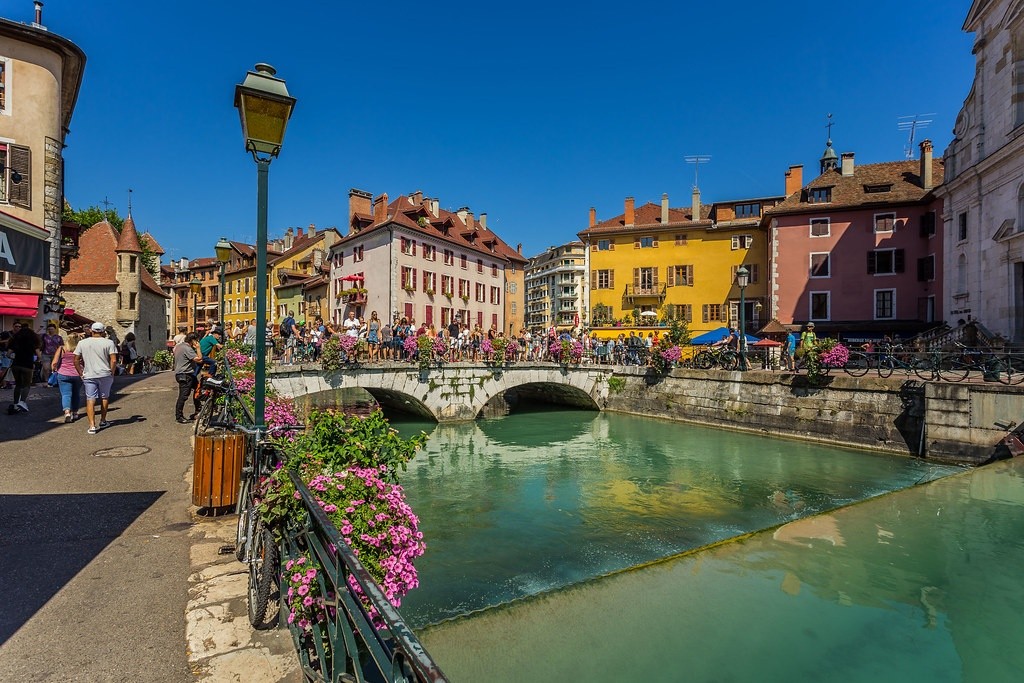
[805,322,814,326]
[211,329,222,335]
[105,326,114,332]
[207,318,214,325]
[92,321,105,332]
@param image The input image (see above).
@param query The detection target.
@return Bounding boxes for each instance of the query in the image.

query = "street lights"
[233,64,299,425]
[214,237,233,345]
[736,264,750,371]
[189,277,202,333]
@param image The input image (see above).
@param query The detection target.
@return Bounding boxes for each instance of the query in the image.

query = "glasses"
[15,326,21,328]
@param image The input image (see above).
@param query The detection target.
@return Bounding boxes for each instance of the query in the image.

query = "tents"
[690,327,762,347]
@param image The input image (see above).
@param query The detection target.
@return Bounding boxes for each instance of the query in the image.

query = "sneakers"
[99,419,110,428]
[13,404,21,412]
[87,427,97,435]
[17,400,29,412]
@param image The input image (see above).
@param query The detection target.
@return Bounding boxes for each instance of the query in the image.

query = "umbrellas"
[639,310,657,326]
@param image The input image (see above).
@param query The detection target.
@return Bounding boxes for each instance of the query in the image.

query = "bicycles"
[206,420,309,627]
[808,346,870,377]
[625,346,645,366]
[196,377,245,440]
[937,341,1024,386]
[694,340,738,371]
[878,344,939,381]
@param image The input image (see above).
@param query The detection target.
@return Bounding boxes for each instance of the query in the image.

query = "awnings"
[0,294,40,318]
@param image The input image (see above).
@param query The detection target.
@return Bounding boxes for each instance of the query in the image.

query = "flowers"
[221,347,424,628]
[805,338,848,377]
[550,339,584,363]
[403,333,446,363]
[318,333,359,368]
[481,337,518,369]
[646,339,685,375]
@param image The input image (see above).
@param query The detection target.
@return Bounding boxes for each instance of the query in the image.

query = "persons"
[1,310,892,434]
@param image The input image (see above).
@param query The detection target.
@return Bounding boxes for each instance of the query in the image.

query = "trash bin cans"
[192,429,246,507]
[983,359,1000,382]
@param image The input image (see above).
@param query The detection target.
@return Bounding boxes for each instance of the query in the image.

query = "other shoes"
[65,413,73,423]
[119,367,125,376]
[178,416,190,422]
[72,413,78,420]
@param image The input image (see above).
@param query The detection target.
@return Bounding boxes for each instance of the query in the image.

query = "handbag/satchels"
[47,371,58,387]
[40,334,46,351]
